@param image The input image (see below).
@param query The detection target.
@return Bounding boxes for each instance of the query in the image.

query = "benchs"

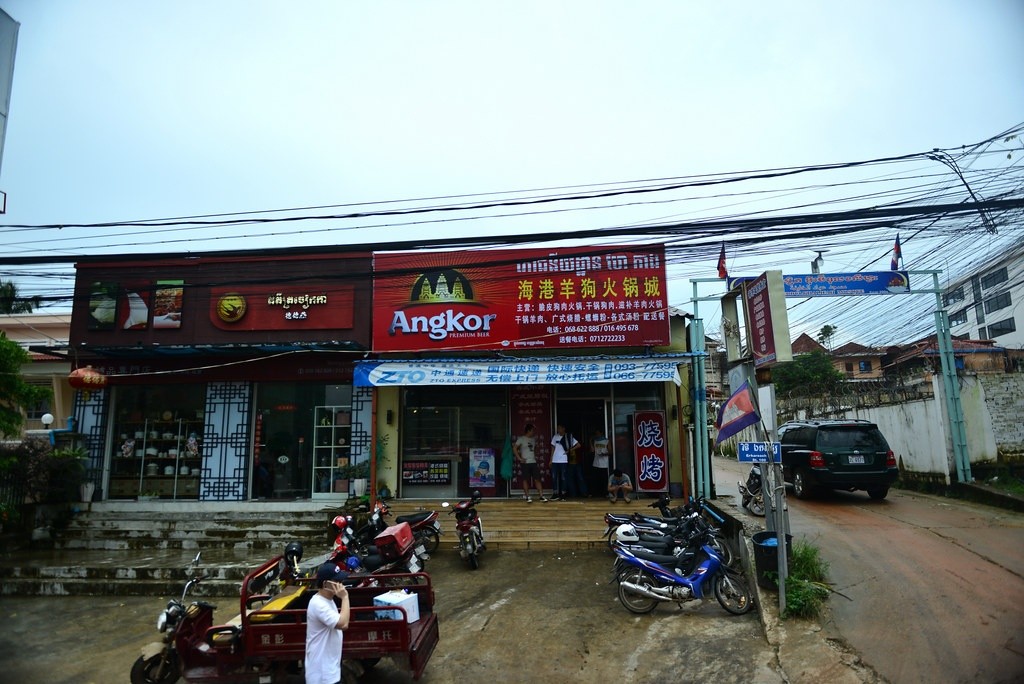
[202,595,272,651]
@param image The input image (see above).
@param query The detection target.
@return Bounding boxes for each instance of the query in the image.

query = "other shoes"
[540,496,548,501]
[611,497,617,503]
[549,496,559,501]
[526,498,532,502]
[561,497,566,501]
[624,497,631,503]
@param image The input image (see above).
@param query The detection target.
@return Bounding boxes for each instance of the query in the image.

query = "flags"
[714,379,760,448]
[891,234,901,270]
[717,244,728,278]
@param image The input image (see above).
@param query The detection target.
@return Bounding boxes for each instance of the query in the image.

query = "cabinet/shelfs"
[311,406,352,500]
[109,418,204,496]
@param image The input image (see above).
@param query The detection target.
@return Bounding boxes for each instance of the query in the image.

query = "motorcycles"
[267,546,380,609]
[328,516,431,574]
[737,463,767,516]
[607,528,751,615]
[601,495,734,583]
[442,490,486,569]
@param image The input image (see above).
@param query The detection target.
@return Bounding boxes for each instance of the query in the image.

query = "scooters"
[130,552,440,683]
[356,496,444,555]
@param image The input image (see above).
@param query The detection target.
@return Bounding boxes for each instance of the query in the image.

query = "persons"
[608,469,633,503]
[589,428,612,498]
[567,448,592,500]
[513,424,548,503]
[548,423,581,501]
[304,563,350,684]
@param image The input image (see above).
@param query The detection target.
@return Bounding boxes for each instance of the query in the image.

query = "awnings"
[351,351,710,386]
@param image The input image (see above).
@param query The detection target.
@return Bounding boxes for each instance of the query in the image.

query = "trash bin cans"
[752,530,793,591]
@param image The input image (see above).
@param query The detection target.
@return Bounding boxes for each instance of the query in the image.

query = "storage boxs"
[373,522,415,561]
[373,591,420,624]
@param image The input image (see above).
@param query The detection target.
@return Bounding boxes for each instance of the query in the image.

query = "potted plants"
[352,461,369,496]
[47,446,93,504]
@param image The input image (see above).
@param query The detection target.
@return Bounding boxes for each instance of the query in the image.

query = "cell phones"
[326,581,337,590]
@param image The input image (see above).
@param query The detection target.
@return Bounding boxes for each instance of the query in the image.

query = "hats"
[317,563,349,581]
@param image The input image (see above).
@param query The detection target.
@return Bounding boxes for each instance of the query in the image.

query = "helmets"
[617,524,639,544]
[285,541,303,564]
[332,516,347,530]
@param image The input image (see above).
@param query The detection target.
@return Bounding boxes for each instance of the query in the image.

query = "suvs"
[777,419,899,500]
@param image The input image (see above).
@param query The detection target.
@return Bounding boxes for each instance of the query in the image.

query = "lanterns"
[67,365,108,401]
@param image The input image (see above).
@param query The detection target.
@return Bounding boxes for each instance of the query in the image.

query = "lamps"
[41,414,53,429]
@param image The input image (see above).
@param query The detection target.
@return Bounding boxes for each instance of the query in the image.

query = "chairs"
[828,432,840,444]
